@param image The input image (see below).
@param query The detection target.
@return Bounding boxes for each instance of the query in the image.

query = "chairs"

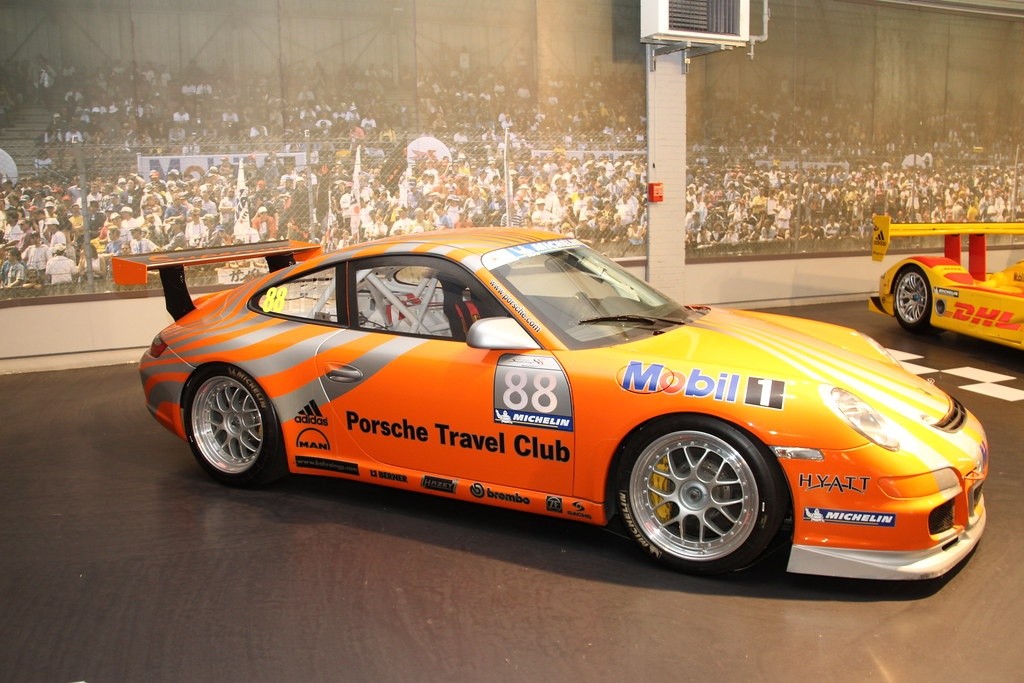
[450,302,496,340]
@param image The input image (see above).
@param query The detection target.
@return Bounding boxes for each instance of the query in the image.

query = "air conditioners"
[639,0,751,43]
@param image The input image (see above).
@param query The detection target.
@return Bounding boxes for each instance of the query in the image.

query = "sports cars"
[112,226,989,581]
[864,213,1022,349]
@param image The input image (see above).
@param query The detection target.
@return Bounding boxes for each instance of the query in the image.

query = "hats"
[201,213,214,220]
[534,197,546,204]
[110,212,120,219]
[686,183,697,191]
[51,244,66,253]
[117,177,126,184]
[119,207,132,212]
[45,201,54,208]
[143,183,156,192]
[45,219,60,225]
[151,173,159,178]
[258,206,267,213]
[128,226,142,231]
[344,182,353,186]
[578,189,584,193]
[429,192,441,197]
[18,195,30,202]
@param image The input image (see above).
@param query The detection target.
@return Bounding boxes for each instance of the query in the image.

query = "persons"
[0,42,1023,289]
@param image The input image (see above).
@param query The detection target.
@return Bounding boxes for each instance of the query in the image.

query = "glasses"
[18,223,23,225]
